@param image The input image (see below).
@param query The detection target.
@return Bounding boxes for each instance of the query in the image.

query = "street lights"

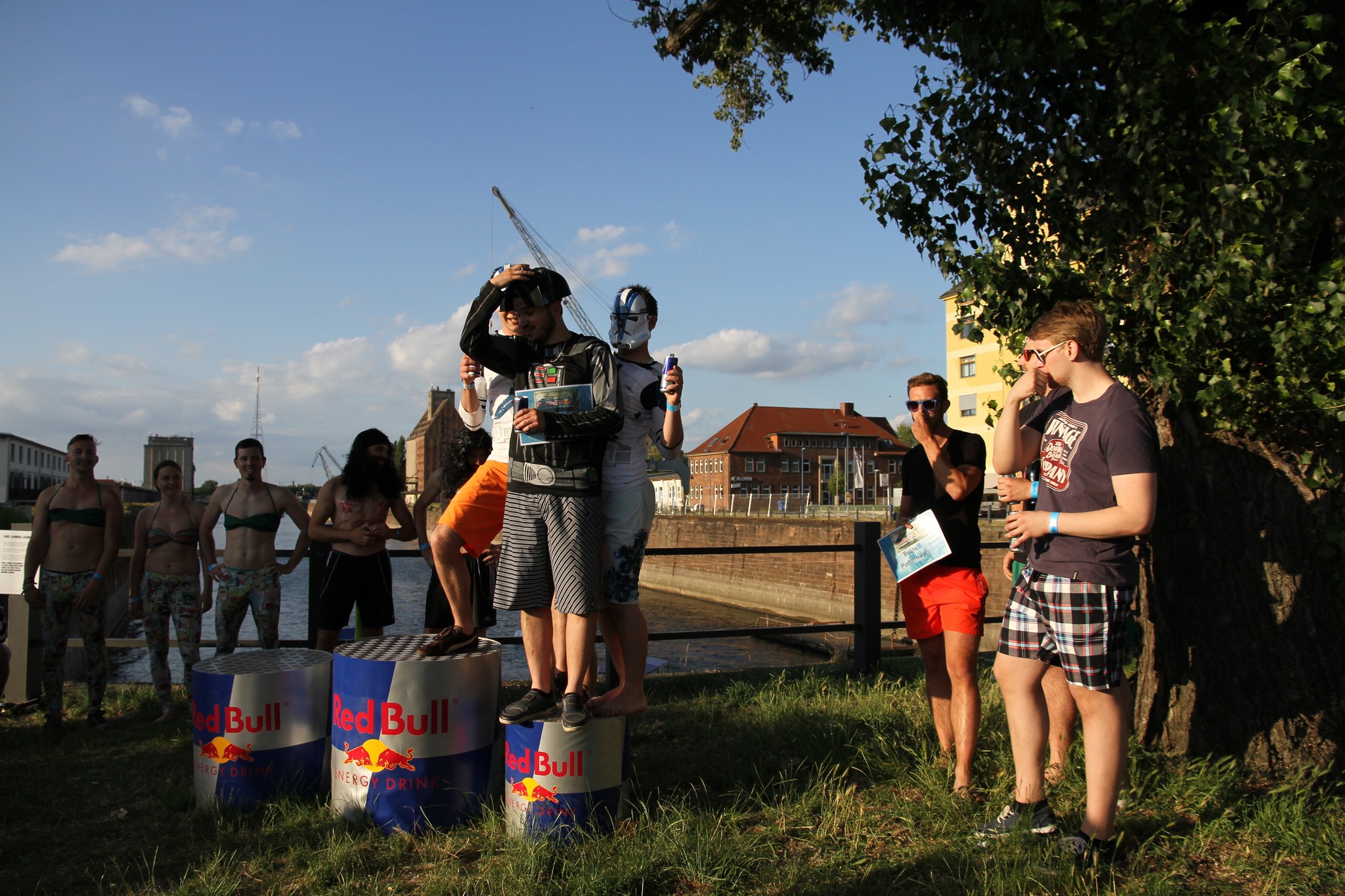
[840,432,850,514]
[801,447,806,516]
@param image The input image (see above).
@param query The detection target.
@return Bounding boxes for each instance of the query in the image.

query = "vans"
[693,503,706,514]
[978,489,1007,519]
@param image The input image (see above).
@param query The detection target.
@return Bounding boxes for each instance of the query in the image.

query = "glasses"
[905,400,945,411]
[1021,350,1035,361]
[1033,341,1068,364]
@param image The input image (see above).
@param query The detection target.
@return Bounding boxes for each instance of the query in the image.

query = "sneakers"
[499,691,558,724]
[553,672,589,705]
[416,625,479,656]
[973,800,1058,840]
[1031,831,1120,880]
[561,695,593,731]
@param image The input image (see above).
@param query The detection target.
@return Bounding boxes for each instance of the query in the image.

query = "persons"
[430,264,571,701]
[0,604,10,707]
[309,428,417,657]
[198,440,314,658]
[887,304,1160,872]
[583,282,684,715]
[129,459,214,725]
[23,432,123,731]
[459,268,626,736]
[410,425,503,644]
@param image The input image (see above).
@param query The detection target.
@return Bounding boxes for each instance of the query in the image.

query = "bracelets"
[394,528,400,540]
[666,400,682,412]
[93,573,105,582]
[1049,511,1058,533]
[128,597,140,604]
[21,577,37,595]
[208,563,218,571]
[1031,481,1040,498]
[461,378,475,389]
[420,543,429,551]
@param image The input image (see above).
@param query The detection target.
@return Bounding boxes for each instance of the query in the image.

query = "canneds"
[468,364,481,377]
[1008,511,1025,552]
[660,353,678,393]
[1002,473,1021,505]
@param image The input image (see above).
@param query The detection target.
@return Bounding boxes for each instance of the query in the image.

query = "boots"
[187,702,193,719]
[152,698,176,724]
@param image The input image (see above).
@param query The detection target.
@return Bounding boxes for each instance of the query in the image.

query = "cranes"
[312,446,344,480]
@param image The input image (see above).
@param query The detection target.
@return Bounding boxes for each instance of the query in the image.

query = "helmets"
[500,267,572,312]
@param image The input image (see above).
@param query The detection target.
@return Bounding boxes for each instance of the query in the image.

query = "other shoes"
[1116,785,1132,812]
[949,785,989,806]
[87,710,110,731]
[930,750,954,771]
[41,713,63,730]
